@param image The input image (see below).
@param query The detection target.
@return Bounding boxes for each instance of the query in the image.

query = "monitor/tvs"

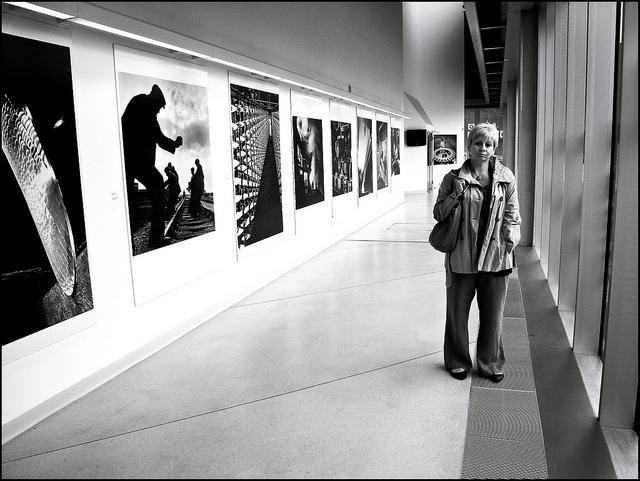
[406,129,427,146]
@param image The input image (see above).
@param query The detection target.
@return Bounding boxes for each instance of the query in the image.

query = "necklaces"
[474,172,482,181]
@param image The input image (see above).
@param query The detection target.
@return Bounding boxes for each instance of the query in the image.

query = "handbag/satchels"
[429,201,462,252]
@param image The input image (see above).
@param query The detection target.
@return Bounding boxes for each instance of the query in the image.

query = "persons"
[122,84,182,247]
[164,159,204,221]
[433,121,521,381]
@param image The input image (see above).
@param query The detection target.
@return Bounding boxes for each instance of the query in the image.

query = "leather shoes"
[487,373,504,382]
[450,367,468,380]
